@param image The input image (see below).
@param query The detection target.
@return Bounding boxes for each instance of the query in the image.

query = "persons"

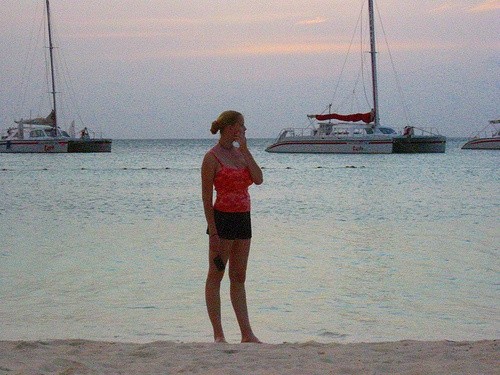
[201,109,264,343]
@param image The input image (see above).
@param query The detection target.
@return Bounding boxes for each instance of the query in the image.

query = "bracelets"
[209,234,218,239]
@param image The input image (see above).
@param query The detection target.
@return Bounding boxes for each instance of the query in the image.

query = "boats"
[460,120,500,151]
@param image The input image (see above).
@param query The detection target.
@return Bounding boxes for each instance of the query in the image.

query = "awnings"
[307,111,374,124]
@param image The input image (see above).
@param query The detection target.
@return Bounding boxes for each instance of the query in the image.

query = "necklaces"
[219,140,233,150]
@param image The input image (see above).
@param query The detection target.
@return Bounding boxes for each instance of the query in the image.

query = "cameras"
[214,255,225,272]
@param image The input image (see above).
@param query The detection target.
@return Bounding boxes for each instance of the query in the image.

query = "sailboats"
[0,0,111,153]
[265,0,446,154]
